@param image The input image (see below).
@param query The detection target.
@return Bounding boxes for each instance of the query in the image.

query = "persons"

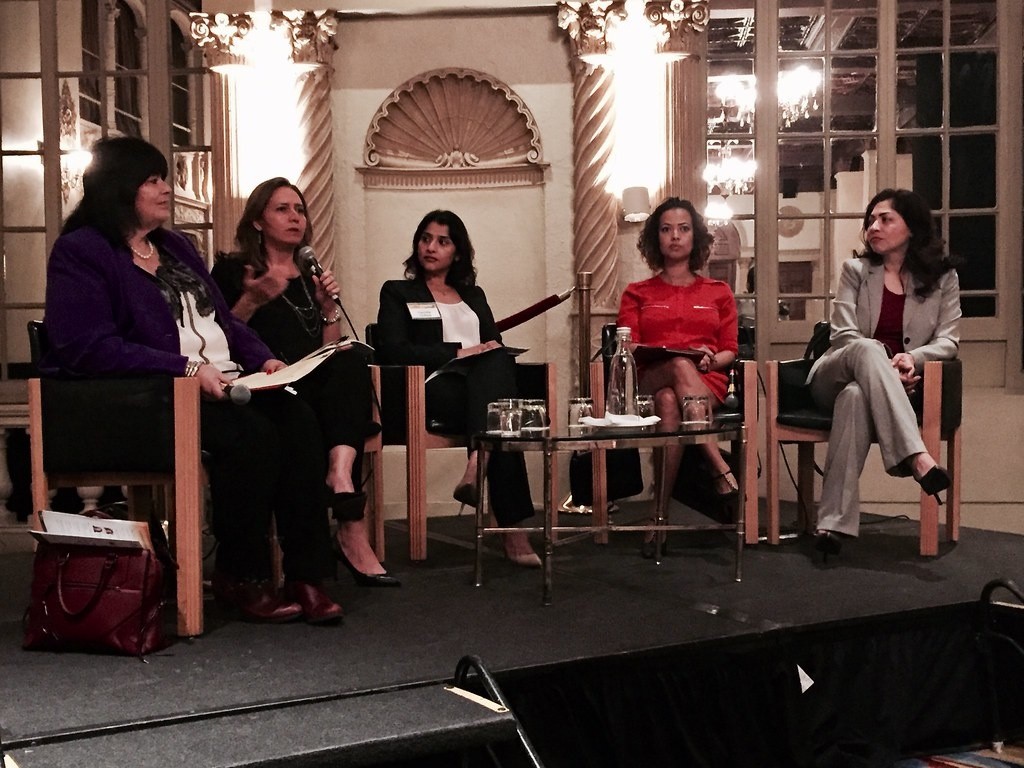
[43,138,342,622]
[804,187,962,569]
[210,178,401,588]
[378,210,540,564]
[105,528,113,534]
[615,197,738,559]
[93,526,101,532]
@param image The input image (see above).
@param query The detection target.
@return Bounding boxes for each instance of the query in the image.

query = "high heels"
[500,533,542,566]
[813,530,840,560]
[920,469,950,505]
[454,484,479,515]
[332,530,402,588]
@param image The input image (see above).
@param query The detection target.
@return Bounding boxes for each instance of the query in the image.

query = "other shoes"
[714,469,740,500]
[644,531,657,560]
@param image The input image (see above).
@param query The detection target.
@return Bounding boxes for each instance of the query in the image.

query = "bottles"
[604,327,639,423]
[724,370,740,415]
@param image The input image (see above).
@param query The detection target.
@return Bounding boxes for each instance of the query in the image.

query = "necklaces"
[438,289,446,295]
[282,262,315,319]
[131,239,153,259]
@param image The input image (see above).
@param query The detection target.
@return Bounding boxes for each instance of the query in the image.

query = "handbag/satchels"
[21,540,169,664]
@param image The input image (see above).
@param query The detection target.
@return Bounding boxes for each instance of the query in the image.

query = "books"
[632,346,706,367]
[229,339,375,390]
[425,346,529,384]
[28,508,154,552]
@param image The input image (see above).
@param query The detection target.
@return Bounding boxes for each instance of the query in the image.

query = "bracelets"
[320,309,341,326]
[185,361,204,376]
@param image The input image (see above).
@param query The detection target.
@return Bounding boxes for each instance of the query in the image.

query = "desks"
[472,423,747,606]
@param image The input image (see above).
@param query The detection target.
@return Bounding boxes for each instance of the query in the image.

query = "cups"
[567,398,595,428]
[682,395,713,424]
[637,395,655,419]
[484,397,547,437]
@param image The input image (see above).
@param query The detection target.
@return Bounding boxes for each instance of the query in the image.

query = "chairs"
[21,317,962,641]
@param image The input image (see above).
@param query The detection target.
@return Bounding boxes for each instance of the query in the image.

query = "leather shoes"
[228,582,302,623]
[285,579,344,621]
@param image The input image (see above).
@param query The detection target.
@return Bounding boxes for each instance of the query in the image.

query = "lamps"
[622,187,650,223]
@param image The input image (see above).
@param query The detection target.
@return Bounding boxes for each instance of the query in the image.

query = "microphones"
[218,383,251,405]
[298,246,341,307]
